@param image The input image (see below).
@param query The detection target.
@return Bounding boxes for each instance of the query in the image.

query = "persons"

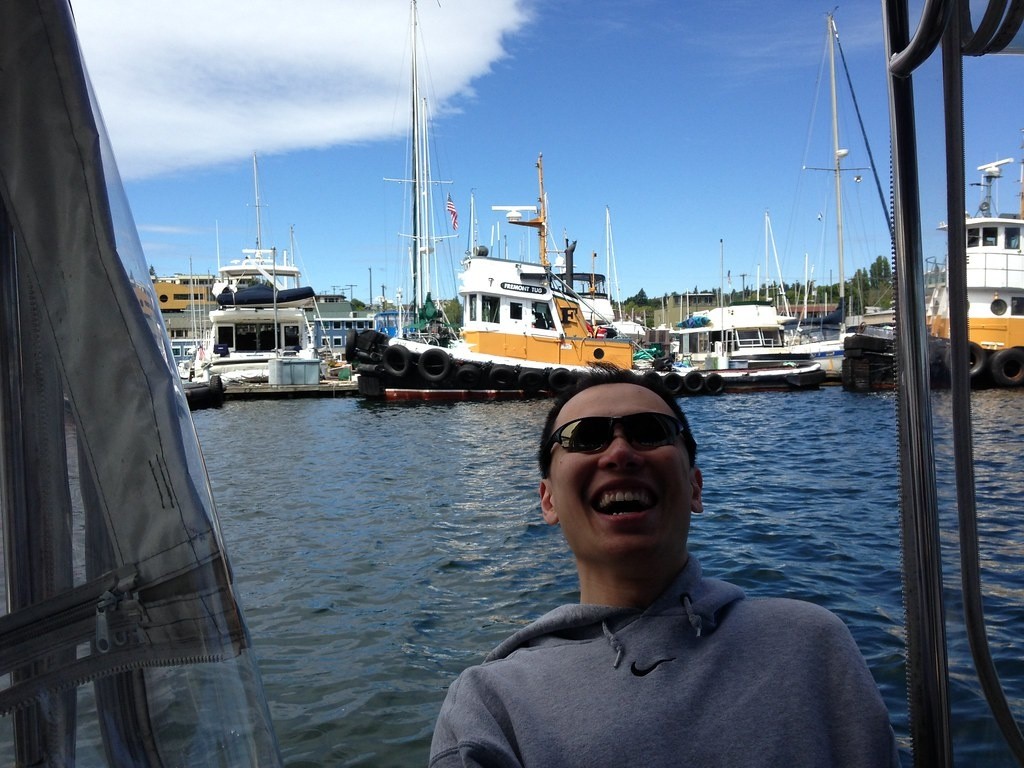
[534,312,547,328]
[429,370,902,767]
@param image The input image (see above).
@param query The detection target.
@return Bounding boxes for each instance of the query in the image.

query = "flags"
[447,193,458,231]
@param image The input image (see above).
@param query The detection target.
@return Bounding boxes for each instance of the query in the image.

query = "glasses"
[545,413,684,453]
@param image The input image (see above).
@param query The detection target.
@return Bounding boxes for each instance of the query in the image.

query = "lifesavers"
[684,372,703,392]
[454,364,475,386]
[706,373,724,393]
[661,373,682,392]
[946,339,984,381]
[341,330,355,356]
[383,344,409,378]
[488,367,515,389]
[517,368,543,392]
[550,368,572,394]
[418,349,452,382]
[641,373,661,391]
[987,346,1024,383]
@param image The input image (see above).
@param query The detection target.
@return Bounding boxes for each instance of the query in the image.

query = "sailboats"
[164,0,1024,406]
